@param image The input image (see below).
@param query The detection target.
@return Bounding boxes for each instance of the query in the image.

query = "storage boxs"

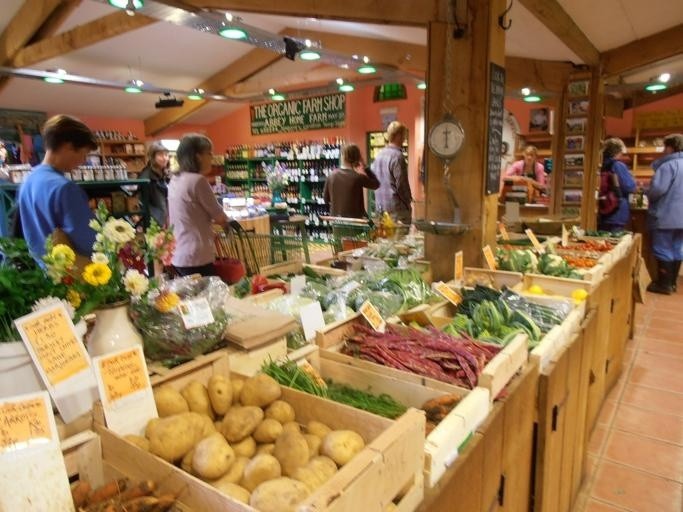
[2,251,424,510]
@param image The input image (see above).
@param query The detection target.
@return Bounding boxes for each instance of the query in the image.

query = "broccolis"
[499,248,579,281]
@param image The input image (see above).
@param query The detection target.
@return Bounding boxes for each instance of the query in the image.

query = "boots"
[647,261,681,295]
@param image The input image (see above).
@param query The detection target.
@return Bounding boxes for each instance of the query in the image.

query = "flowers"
[0,199,176,339]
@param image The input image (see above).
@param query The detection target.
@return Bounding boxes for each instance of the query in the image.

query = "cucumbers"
[455,284,562,331]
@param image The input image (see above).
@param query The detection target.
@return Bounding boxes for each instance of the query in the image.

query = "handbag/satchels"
[212,258,244,283]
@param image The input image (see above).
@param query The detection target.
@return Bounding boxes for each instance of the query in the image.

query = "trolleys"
[323,213,408,257]
[208,214,310,278]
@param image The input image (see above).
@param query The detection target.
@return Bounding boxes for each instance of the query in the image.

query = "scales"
[415,93,471,235]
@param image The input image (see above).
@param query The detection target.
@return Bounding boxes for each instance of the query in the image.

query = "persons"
[646,133,683,294]
[137,133,235,277]
[531,109,547,127]
[599,137,636,232]
[503,145,547,194]
[323,142,380,218]
[371,121,413,223]
[18,114,102,272]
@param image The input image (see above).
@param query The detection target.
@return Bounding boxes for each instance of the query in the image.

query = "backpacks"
[598,168,622,217]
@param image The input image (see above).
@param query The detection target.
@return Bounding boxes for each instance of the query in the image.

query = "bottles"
[226,136,342,245]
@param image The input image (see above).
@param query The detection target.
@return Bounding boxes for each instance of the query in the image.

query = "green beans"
[340,298,541,391]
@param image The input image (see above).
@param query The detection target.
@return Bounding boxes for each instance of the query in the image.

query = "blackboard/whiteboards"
[485,62,506,195]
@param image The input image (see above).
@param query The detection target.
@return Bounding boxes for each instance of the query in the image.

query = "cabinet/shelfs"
[1,77,683,249]
[424,232,641,510]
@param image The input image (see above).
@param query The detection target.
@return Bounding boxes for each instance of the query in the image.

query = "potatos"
[125,371,366,511]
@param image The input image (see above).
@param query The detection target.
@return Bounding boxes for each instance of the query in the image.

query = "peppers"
[248,274,290,294]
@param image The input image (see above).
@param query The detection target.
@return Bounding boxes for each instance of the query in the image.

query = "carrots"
[400,394,462,438]
[74,481,189,511]
